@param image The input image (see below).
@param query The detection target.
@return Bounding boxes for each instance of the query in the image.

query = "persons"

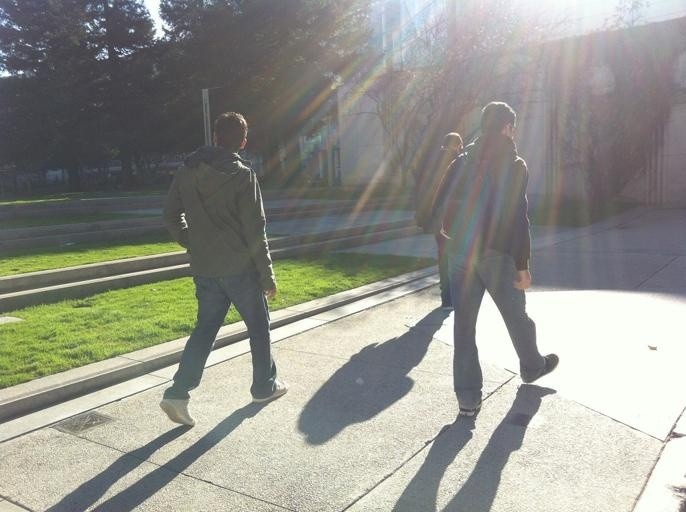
[432,99,562,421]
[157,110,291,426]
[416,131,466,314]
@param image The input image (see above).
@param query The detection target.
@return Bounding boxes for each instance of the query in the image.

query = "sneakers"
[159,398,195,427]
[252,379,289,404]
[540,352,559,378]
[458,397,483,418]
